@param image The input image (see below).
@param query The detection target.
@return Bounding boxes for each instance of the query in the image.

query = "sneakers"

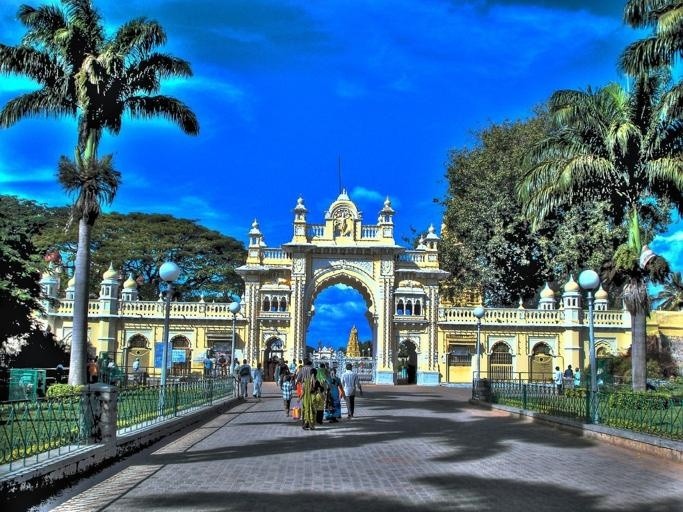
[302,423,314,430]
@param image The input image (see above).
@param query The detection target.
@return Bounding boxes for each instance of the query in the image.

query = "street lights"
[227,300,244,378]
[577,269,605,424]
[155,258,181,417]
[470,304,486,396]
[361,348,365,356]
[367,347,371,356]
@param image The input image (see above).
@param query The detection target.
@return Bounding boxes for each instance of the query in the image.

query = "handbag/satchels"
[293,407,301,419]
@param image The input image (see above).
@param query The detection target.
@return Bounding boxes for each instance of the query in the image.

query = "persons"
[106,358,117,369]
[563,364,573,378]
[131,357,139,371]
[238,358,251,397]
[252,362,264,399]
[553,366,563,395]
[201,354,239,377]
[272,357,363,431]
[54,360,63,383]
[573,367,580,387]
